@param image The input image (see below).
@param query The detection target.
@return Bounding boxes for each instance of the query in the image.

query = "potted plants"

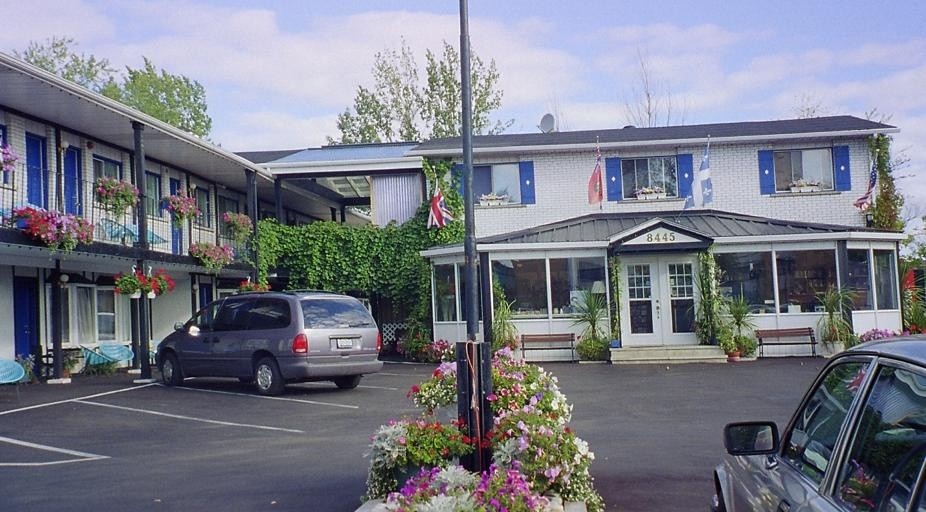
[813,285,861,356]
[634,186,667,199]
[573,288,613,361]
[787,178,823,193]
[478,191,512,206]
[692,245,759,363]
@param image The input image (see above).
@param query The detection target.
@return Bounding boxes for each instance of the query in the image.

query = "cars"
[714,334,926,512]
[155,289,384,396]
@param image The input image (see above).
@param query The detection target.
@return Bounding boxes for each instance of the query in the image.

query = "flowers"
[93,177,272,294]
[397,334,455,360]
[0,143,20,172]
[356,353,608,512]
[6,205,96,258]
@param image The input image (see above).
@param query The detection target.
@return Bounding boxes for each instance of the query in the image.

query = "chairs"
[74,344,135,378]
[0,358,26,407]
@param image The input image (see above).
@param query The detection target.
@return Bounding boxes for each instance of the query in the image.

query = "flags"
[426,186,454,229]
[853,159,878,211]
[681,147,714,210]
[588,156,604,205]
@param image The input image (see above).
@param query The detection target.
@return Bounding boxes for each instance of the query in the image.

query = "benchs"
[518,333,576,360]
[754,327,819,359]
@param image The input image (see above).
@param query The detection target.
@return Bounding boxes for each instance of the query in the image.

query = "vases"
[129,288,156,299]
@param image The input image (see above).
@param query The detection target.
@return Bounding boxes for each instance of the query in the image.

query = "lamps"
[590,280,606,306]
[865,213,873,225]
